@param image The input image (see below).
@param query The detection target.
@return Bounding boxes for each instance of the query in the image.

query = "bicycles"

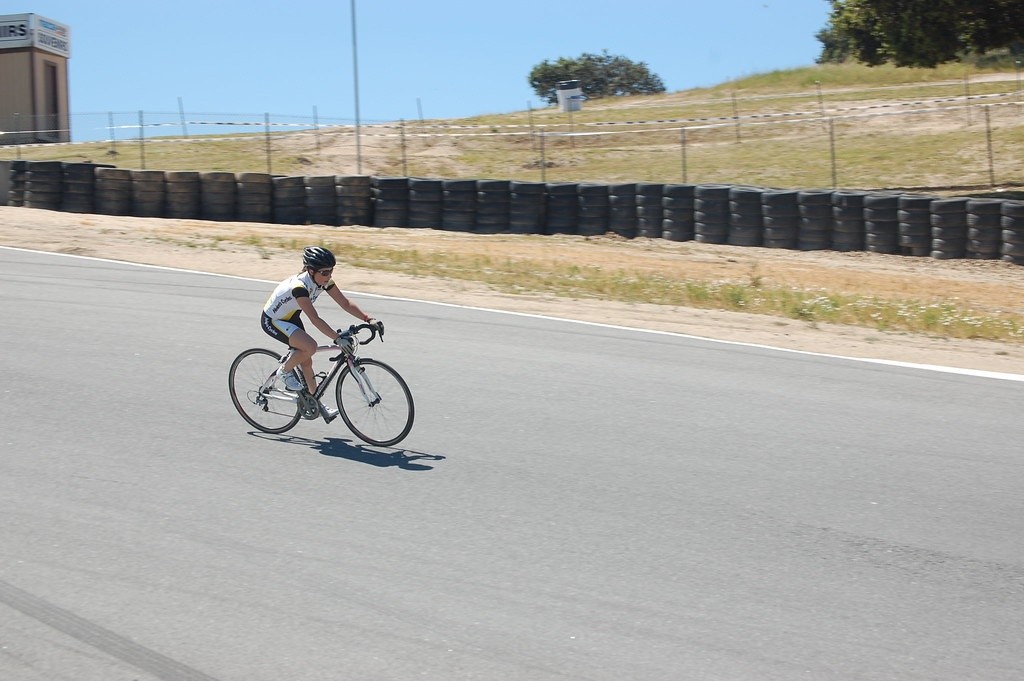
[228,322,414,447]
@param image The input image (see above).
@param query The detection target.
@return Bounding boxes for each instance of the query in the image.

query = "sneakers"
[318,404,339,418]
[276,366,305,390]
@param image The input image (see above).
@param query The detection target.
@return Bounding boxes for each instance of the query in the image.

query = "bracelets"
[364,314,368,321]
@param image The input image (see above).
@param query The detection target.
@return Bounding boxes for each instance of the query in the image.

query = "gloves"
[335,337,354,356]
[368,319,384,336]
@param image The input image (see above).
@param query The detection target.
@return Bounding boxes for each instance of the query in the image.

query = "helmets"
[302,247,336,270]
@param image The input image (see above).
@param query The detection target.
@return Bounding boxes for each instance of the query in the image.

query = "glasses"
[313,268,333,276]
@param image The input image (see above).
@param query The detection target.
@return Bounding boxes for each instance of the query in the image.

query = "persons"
[259,245,381,419]
